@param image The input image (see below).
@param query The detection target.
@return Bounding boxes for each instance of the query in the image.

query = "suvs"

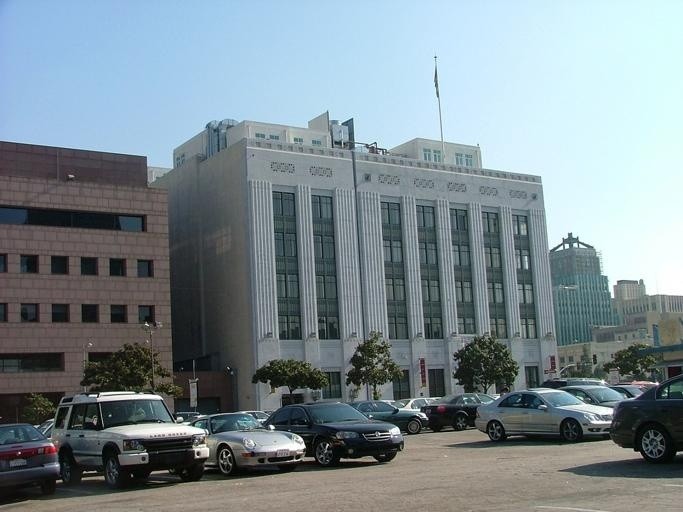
[50,390,210,489]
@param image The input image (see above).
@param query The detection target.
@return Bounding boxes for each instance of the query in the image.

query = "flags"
[434,59,439,98]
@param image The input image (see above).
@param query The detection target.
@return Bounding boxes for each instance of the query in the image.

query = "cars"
[0,417,68,495]
[170,403,307,476]
[260,398,404,469]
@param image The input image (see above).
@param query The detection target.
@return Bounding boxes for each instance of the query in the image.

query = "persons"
[500,387,509,396]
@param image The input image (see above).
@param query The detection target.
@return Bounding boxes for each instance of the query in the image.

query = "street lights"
[141,321,164,393]
[82,342,95,392]
